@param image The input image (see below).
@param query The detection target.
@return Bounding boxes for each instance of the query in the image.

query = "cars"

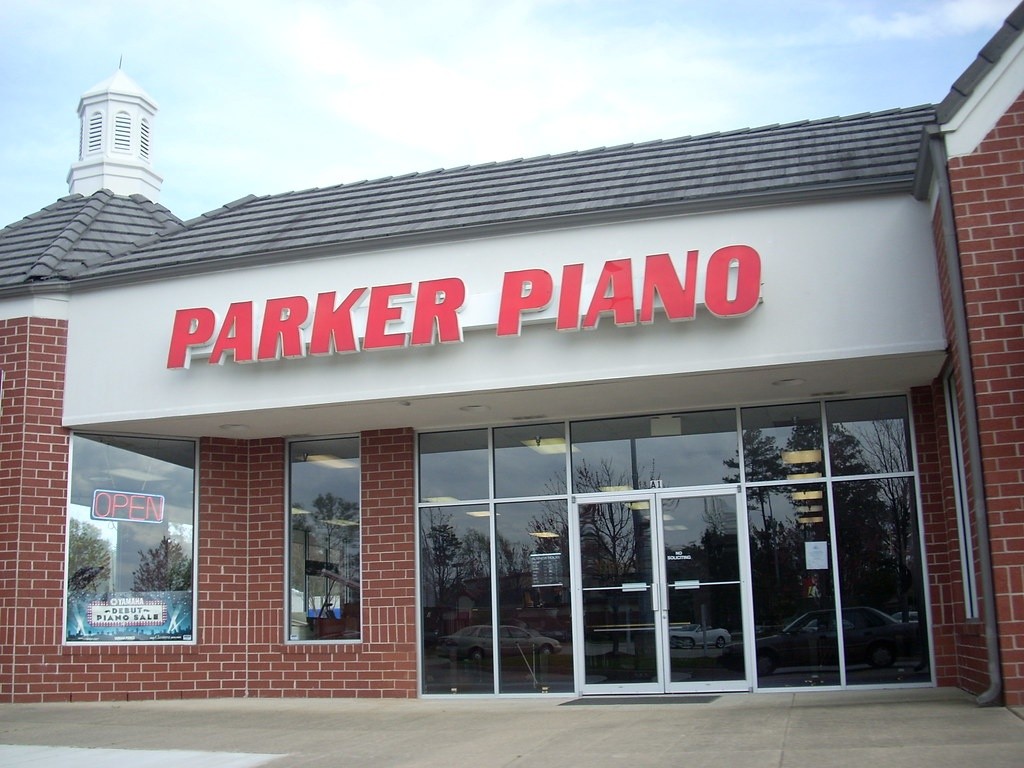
[720,607,919,676]
[892,612,918,623]
[437,625,563,659]
[670,623,731,650]
